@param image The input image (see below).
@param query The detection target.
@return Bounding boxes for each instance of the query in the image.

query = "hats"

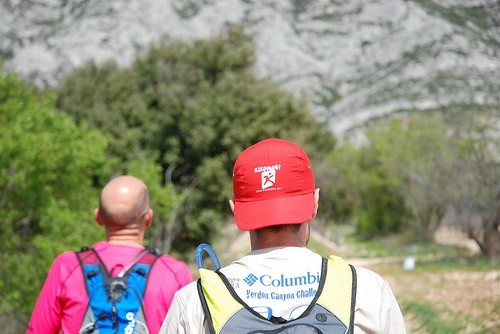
[233,136,315,229]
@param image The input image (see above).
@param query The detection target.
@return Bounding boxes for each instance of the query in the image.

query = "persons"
[159,138,407,334]
[25,175,193,334]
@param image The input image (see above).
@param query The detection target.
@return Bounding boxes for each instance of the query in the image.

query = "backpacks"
[197,255,356,334]
[75,244,164,334]
